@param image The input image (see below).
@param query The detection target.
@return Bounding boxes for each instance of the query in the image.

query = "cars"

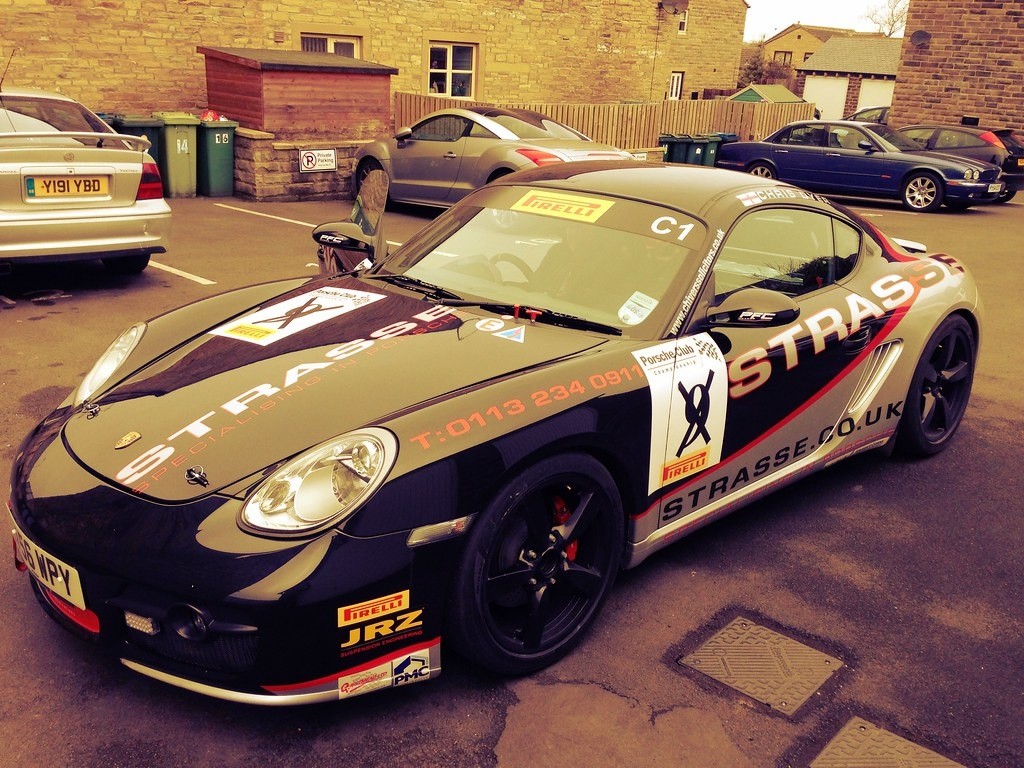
[0,87,177,276]
[713,117,1006,213]
[838,103,890,125]
[348,105,635,212]
[880,122,1024,204]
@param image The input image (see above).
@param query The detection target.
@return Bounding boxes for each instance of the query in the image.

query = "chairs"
[317,167,391,274]
[790,128,842,148]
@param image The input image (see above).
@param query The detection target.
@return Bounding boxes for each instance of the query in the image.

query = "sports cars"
[6,154,989,714]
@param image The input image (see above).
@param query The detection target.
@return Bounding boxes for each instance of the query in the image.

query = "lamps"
[961,115,980,127]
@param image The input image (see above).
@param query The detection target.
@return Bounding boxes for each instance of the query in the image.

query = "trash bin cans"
[658,133,692,164]
[113,113,165,167]
[716,133,742,162]
[682,134,710,166]
[96,111,123,135]
[149,110,201,198]
[697,134,722,167]
[194,116,240,197]
